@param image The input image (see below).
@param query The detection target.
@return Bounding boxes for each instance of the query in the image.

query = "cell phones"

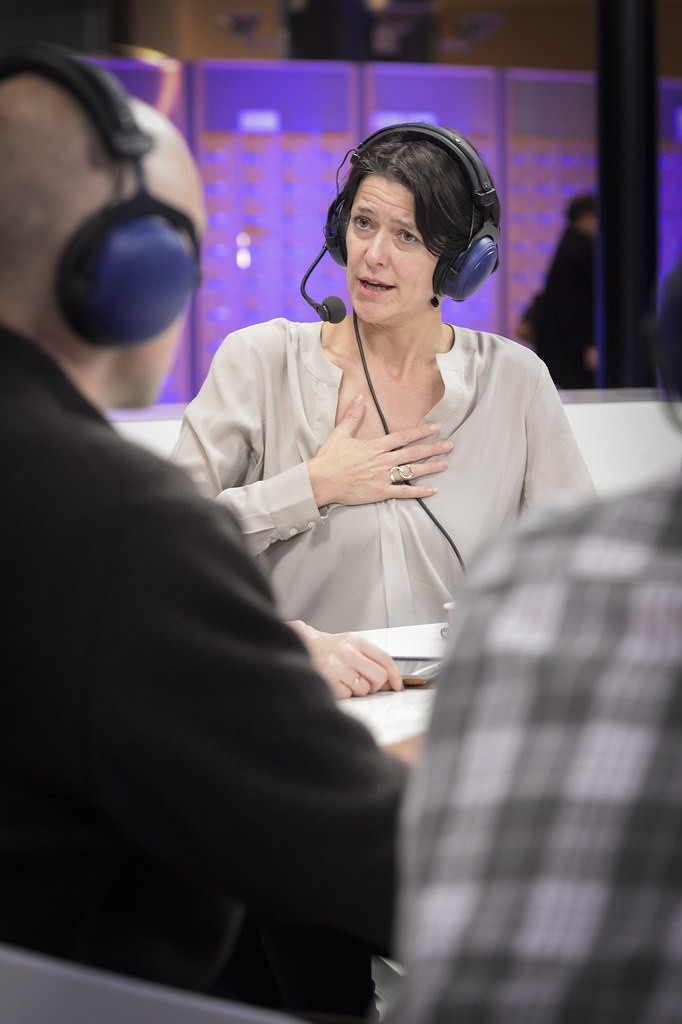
[390,656,448,685]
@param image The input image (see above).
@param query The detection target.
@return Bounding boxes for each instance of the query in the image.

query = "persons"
[534,197,603,389]
[284,620,403,698]
[168,122,599,630]
[387,264,682,1024]
[1,37,416,1023]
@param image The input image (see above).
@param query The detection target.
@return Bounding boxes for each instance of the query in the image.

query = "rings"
[351,674,362,687]
[390,464,415,484]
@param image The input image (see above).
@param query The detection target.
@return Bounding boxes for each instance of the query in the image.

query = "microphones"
[301,241,346,325]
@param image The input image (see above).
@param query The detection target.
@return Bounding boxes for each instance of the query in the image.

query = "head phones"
[325,121,500,302]
[0,38,202,348]
